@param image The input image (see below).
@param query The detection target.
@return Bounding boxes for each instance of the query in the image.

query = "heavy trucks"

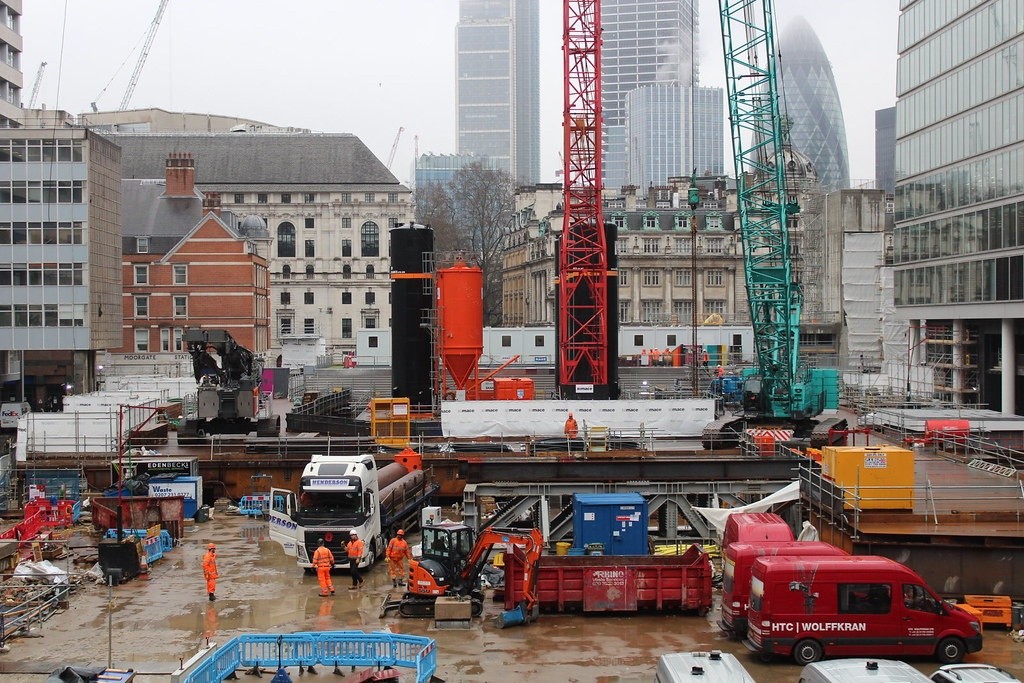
[269,454,435,574]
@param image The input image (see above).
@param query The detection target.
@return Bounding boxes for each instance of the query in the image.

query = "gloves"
[356,559,360,564]
[409,558,414,562]
[311,567,315,572]
[330,564,334,569]
[341,541,346,549]
[384,558,390,563]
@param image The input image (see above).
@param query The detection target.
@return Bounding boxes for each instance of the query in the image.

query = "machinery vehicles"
[378,514,543,629]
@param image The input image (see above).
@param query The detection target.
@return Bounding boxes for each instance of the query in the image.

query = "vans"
[723,513,795,542]
[742,556,982,663]
[717,541,851,639]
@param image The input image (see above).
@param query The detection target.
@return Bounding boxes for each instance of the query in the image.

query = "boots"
[399,579,406,586]
[210,593,216,601]
[393,579,397,587]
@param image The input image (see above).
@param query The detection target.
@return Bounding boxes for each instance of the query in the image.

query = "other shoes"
[348,583,358,589]
[319,593,327,596]
[360,581,365,587]
[331,590,335,594]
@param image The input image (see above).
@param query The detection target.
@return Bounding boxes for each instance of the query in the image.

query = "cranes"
[386,125,406,170]
[29,61,47,109]
[92,0,167,112]
[717,0,839,419]
[561,0,612,399]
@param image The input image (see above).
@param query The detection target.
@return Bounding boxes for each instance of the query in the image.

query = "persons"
[343,530,366,592]
[717,364,724,377]
[564,412,579,440]
[385,528,414,587]
[642,346,673,367]
[202,541,221,602]
[311,538,336,596]
[703,349,711,368]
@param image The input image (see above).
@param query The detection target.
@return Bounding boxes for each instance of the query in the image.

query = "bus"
[239,496,285,517]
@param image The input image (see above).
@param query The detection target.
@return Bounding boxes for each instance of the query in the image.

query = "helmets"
[666,348,670,351]
[397,529,404,536]
[569,413,573,417]
[654,347,658,351]
[650,347,652,350]
[703,349,707,352]
[349,530,357,535]
[642,348,646,352]
[316,539,324,544]
[207,543,216,549]
[717,365,721,367]
